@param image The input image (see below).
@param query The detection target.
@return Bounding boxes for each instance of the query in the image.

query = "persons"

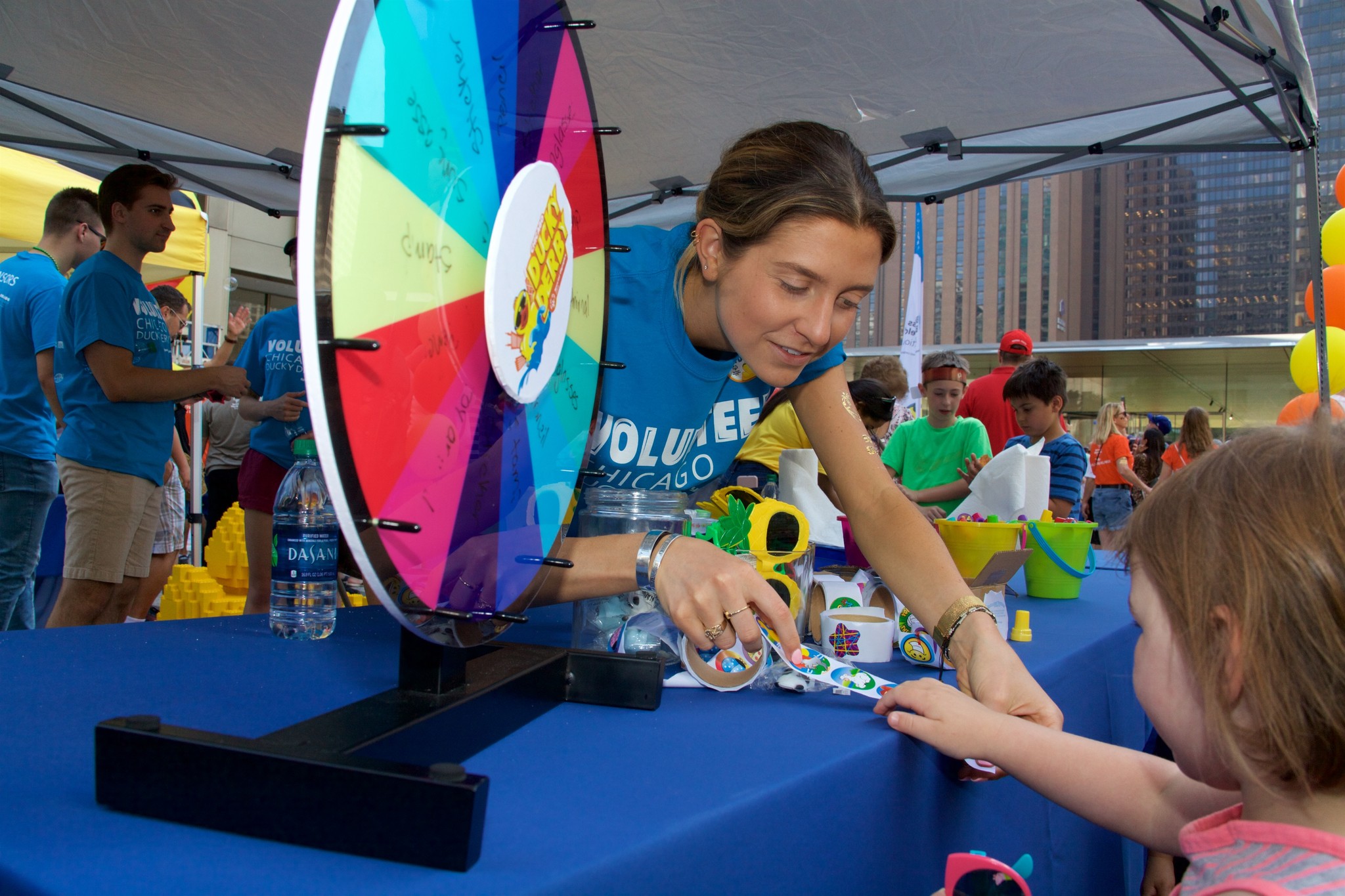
[1329,389,1345,410]
[718,330,1224,549]
[0,160,384,629]
[429,524,558,614]
[504,119,1056,784]
[870,424,1345,896]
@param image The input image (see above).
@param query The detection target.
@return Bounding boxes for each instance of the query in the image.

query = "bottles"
[578,486,694,666]
[268,438,340,639]
[690,508,721,546]
[760,473,779,501]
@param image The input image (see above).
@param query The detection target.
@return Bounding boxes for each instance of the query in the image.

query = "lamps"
[1209,400,1214,406]
[1229,414,1233,420]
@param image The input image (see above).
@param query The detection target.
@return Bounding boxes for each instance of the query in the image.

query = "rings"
[477,598,493,612]
[703,618,727,641]
[724,605,750,617]
[458,576,476,591]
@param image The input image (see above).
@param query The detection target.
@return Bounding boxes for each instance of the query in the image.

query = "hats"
[283,235,297,256]
[999,329,1033,355]
[1147,413,1172,436]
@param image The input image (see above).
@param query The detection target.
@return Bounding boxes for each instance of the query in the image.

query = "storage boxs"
[962,548,1035,603]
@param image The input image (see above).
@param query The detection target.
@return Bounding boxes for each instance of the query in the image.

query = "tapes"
[804,572,845,634]
[808,583,863,644]
[862,581,921,650]
[851,570,874,595]
[898,608,956,672]
[819,606,895,663]
[680,603,996,775]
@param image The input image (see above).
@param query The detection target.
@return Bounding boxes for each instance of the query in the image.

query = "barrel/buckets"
[933,518,1026,588]
[1019,518,1098,600]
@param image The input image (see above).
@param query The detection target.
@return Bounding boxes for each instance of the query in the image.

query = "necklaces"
[32,246,61,272]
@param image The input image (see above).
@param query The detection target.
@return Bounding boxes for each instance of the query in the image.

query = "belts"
[1096,484,1130,491]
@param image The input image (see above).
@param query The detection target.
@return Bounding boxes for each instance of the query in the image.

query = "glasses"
[696,485,765,520]
[944,850,1034,896]
[169,308,188,330]
[743,496,811,621]
[76,219,107,251]
[1113,412,1127,417]
[863,396,896,407]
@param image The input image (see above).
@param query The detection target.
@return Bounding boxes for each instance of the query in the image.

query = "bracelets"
[933,595,998,685]
[636,529,684,593]
[224,335,237,344]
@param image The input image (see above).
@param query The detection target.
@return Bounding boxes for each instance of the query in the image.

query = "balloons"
[1334,163,1345,208]
[1305,265,1345,331]
[1290,326,1345,397]
[1320,208,1345,266]
[1276,393,1345,426]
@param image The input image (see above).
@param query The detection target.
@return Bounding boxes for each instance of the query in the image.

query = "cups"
[735,541,815,645]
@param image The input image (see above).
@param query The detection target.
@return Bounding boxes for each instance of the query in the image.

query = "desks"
[0,550,1156,896]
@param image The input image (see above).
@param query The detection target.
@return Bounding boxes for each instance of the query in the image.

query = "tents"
[0,143,210,567]
[1,1,1330,408]
